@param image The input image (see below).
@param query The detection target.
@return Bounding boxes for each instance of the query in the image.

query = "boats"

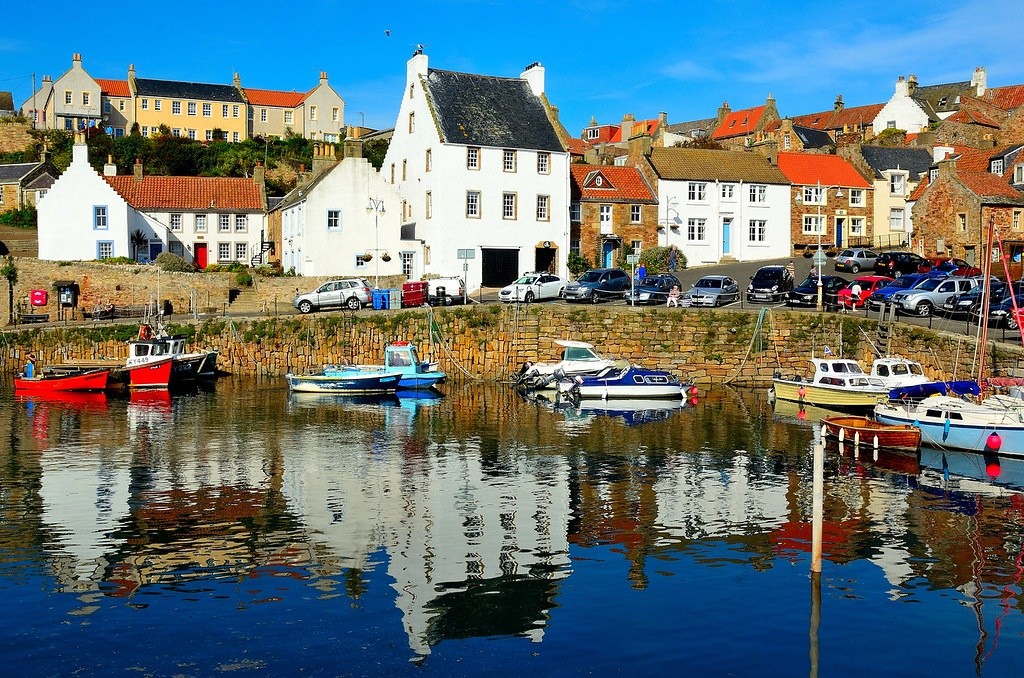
[321,339,448,390]
[383,386,447,438]
[819,438,921,478]
[13,388,110,410]
[513,385,689,426]
[106,387,172,406]
[509,340,699,397]
[771,325,929,406]
[774,398,854,429]
[818,414,923,451]
[287,392,402,412]
[13,262,218,391]
[285,370,404,393]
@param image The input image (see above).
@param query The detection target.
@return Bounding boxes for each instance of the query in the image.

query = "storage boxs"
[371,282,428,310]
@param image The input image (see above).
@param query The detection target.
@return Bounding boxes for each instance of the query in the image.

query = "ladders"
[874,303,896,360]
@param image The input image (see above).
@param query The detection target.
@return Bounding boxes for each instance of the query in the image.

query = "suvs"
[561,268,631,305]
[868,263,959,313]
[943,281,1023,322]
[426,275,468,306]
[293,277,373,315]
[917,256,982,276]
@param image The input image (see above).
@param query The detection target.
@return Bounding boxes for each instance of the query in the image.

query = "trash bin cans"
[390,289,401,309]
[371,289,390,310]
[402,282,429,308]
[436,286,446,306]
[826,289,839,312]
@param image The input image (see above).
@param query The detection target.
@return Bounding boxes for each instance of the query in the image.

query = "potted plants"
[360,252,373,262]
[380,252,391,262]
[802,246,813,258]
[825,244,838,257]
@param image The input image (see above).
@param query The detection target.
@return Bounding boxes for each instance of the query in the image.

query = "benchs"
[21,314,49,323]
[80,310,112,321]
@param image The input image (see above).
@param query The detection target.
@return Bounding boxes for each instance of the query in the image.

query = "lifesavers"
[391,341,409,346]
[140,326,151,340]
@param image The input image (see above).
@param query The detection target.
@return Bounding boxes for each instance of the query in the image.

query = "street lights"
[365,195,387,291]
[795,179,845,313]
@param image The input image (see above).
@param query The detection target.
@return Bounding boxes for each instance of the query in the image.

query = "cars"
[834,248,878,274]
[682,275,740,307]
[498,272,571,304]
[783,275,852,308]
[746,265,794,305]
[969,293,1024,330]
[872,250,924,279]
[837,276,901,310]
[624,272,682,305]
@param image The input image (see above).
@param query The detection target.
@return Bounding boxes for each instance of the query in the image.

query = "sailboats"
[921,444,1024,678]
[873,208,1024,461]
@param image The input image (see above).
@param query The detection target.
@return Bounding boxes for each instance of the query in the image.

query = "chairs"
[335,282,343,290]
[664,289,683,307]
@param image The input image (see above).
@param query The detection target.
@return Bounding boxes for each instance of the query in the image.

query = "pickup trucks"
[888,275,1004,318]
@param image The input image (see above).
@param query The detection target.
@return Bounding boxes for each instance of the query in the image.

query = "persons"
[25,352,37,378]
[810,265,816,275]
[667,286,678,307]
[639,263,646,279]
[667,246,678,272]
[851,282,861,312]
[786,261,794,277]
[92,300,113,321]
